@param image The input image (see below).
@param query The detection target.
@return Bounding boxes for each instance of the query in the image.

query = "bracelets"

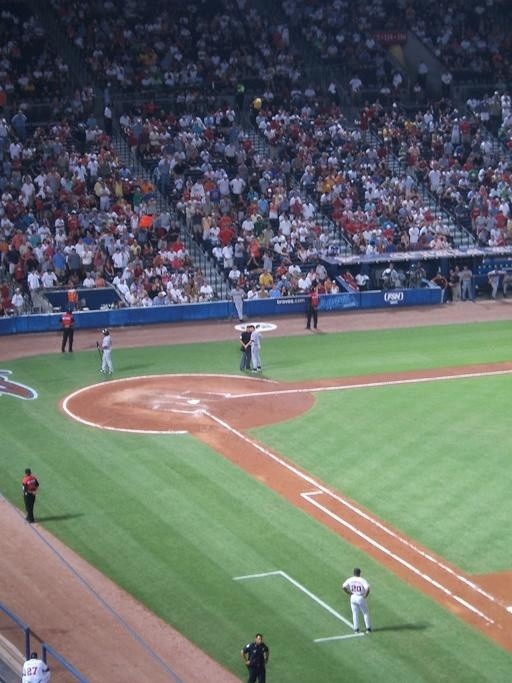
[266,658,268,661]
[98,346,99,349]
[348,592,350,595]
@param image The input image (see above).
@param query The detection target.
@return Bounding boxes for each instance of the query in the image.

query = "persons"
[22,468,39,524]
[97,328,113,375]
[239,326,253,372]
[59,309,75,353]
[342,568,371,634]
[306,287,319,329]
[21,652,51,683]
[0,0,512,315]
[240,633,269,683]
[245,325,262,374]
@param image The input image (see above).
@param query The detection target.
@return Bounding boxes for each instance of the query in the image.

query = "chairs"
[1,1,511,316]
